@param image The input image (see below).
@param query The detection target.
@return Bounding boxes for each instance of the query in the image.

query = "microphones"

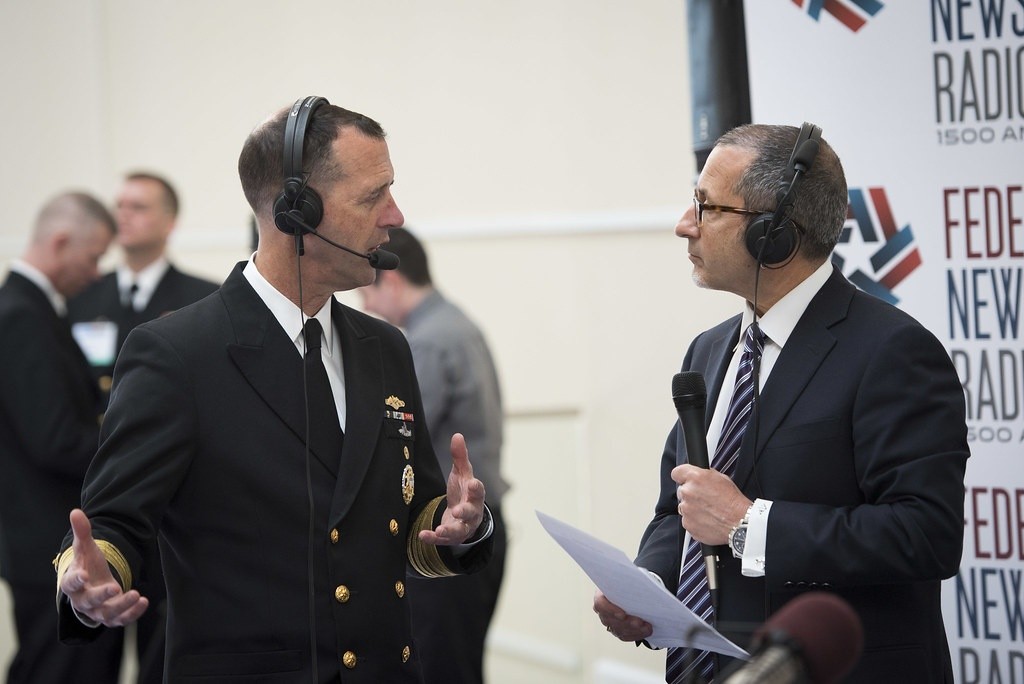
[721,595,862,684]
[672,371,720,610]
[772,139,820,226]
[316,232,399,270]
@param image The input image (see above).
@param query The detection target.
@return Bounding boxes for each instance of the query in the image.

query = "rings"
[607,626,611,632]
[461,521,470,536]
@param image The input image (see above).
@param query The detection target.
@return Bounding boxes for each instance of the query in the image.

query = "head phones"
[743,121,823,262]
[272,96,330,256]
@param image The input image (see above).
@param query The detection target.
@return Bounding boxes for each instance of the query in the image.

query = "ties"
[127,284,138,309]
[666,321,764,684]
[300,318,338,429]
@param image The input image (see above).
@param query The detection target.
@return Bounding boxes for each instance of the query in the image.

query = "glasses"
[692,185,805,234]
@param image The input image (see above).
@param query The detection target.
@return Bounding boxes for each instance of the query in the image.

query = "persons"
[0,191,132,684]
[362,224,509,684]
[593,120,971,684]
[51,96,495,684]
[63,173,224,684]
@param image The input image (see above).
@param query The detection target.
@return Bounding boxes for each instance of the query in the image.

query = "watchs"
[728,501,756,559]
